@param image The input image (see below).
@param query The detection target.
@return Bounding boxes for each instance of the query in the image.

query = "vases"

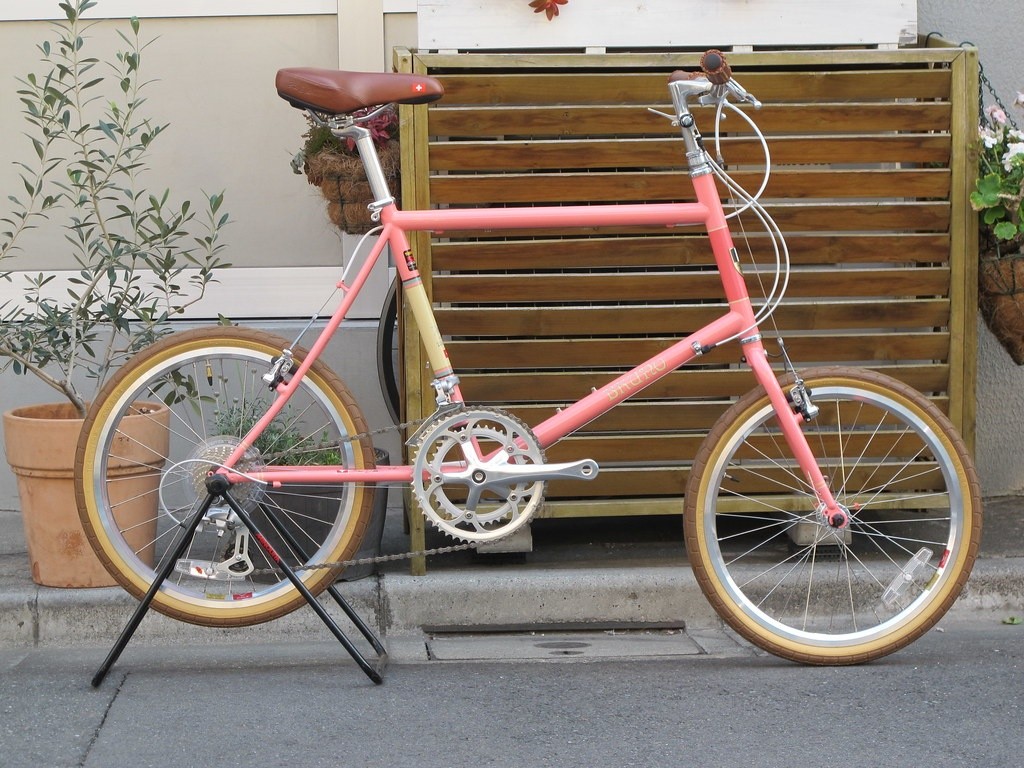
[977,229,1024,366]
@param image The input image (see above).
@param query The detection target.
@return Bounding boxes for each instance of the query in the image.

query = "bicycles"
[73,49,984,689]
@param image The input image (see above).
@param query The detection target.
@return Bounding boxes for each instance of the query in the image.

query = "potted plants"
[217,396,392,582]
[0,1,229,586]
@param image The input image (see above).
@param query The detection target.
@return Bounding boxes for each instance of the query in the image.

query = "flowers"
[928,94,1024,251]
